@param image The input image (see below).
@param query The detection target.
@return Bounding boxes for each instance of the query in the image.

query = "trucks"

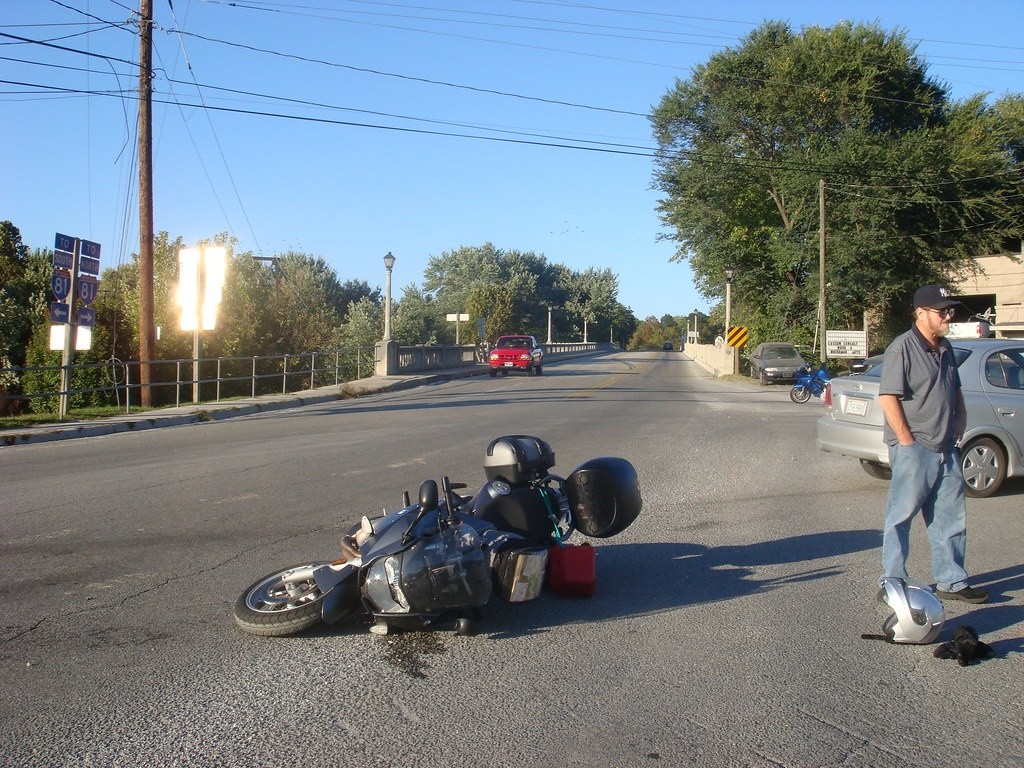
[943,322,991,339]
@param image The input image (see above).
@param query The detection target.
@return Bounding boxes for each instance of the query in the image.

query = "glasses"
[923,308,955,319]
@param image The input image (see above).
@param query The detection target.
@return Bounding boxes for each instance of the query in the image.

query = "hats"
[913,286,961,309]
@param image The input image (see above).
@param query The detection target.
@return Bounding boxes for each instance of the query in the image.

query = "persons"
[875,283,990,604]
[505,340,512,347]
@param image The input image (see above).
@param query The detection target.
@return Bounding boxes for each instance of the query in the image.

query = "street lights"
[381,251,396,343]
[686,319,690,343]
[693,308,698,344]
[610,325,613,343]
[546,304,552,344]
[583,315,588,343]
[723,264,735,345]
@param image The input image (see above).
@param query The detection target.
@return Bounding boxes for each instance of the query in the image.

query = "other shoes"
[877,579,906,602]
[935,585,991,604]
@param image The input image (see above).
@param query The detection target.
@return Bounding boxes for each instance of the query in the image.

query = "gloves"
[933,626,995,667]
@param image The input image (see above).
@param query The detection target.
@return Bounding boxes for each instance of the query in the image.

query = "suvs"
[488,335,543,377]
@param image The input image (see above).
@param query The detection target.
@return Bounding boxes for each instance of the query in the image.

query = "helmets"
[883,578,945,644]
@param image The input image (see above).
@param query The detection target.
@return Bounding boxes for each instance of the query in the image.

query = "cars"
[815,338,1024,499]
[663,342,673,351]
[749,342,807,386]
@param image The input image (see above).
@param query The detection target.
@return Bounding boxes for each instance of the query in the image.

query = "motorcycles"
[233,434,643,637]
[790,361,865,404]
